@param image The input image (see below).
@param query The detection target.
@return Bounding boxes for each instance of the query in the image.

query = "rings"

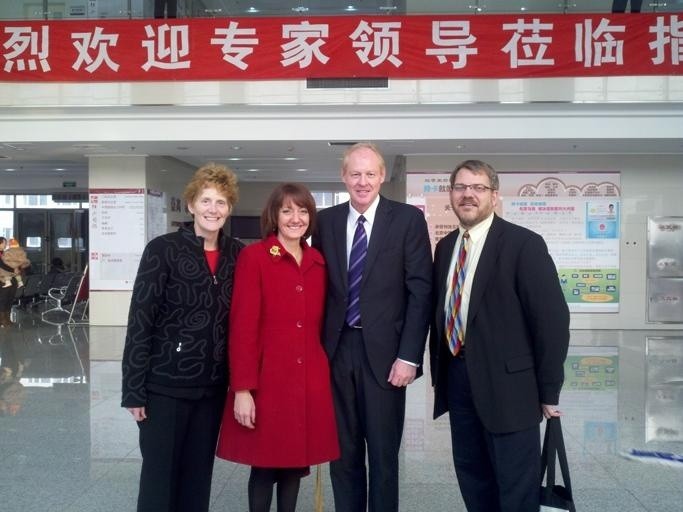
[403,380,408,385]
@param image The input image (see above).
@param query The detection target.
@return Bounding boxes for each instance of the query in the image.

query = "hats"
[8,238,19,248]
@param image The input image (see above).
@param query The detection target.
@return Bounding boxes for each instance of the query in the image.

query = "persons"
[0,236,20,328]
[121,163,246,511]
[1,360,27,414]
[311,143,436,511]
[154,0,177,18]
[611,1,642,14]
[0,329,31,416]
[0,239,29,288]
[217,183,341,511]
[14,257,62,312]
[426,159,570,511]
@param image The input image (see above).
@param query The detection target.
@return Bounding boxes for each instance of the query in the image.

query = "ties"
[444,232,470,356]
[346,214,369,327]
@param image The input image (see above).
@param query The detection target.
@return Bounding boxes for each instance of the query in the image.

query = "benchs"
[9,270,84,319]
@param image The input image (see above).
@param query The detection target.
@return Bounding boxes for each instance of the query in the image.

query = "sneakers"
[2,281,24,289]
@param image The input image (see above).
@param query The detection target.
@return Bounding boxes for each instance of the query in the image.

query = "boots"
[0,311,14,328]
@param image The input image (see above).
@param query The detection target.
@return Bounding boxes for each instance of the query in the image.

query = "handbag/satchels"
[539,417,576,512]
